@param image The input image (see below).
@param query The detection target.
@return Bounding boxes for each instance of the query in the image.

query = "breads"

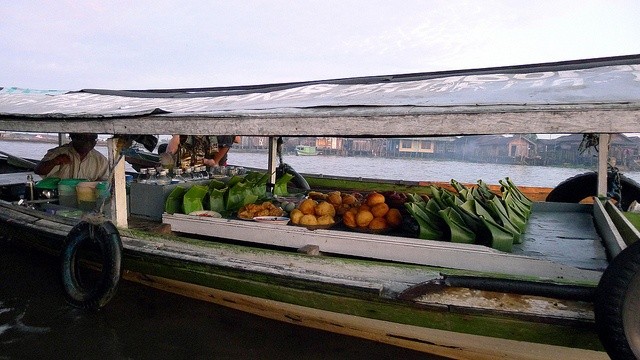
[290,198,336,225]
[309,190,363,216]
[342,192,401,229]
[239,202,285,219]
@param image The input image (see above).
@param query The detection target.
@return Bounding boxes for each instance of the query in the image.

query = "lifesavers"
[275,165,311,191]
[593,239,639,360]
[545,172,640,206]
[59,221,124,310]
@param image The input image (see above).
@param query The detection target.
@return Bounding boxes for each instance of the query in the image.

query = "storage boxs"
[130,174,251,222]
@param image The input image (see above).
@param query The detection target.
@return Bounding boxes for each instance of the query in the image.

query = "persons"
[34,133,110,181]
[158,134,241,170]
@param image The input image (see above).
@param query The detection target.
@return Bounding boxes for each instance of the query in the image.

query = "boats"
[295,144,323,156]
[0,53,639,360]
